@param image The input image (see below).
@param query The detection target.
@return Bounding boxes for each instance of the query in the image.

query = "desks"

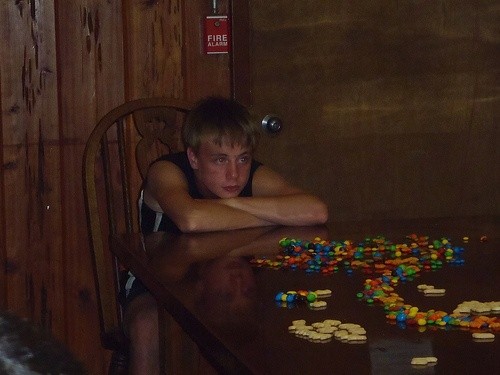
[108,212,500,373]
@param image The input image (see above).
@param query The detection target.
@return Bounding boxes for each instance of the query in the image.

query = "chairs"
[82,97,219,374]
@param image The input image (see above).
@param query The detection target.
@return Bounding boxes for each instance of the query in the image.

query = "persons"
[112,96,330,375]
